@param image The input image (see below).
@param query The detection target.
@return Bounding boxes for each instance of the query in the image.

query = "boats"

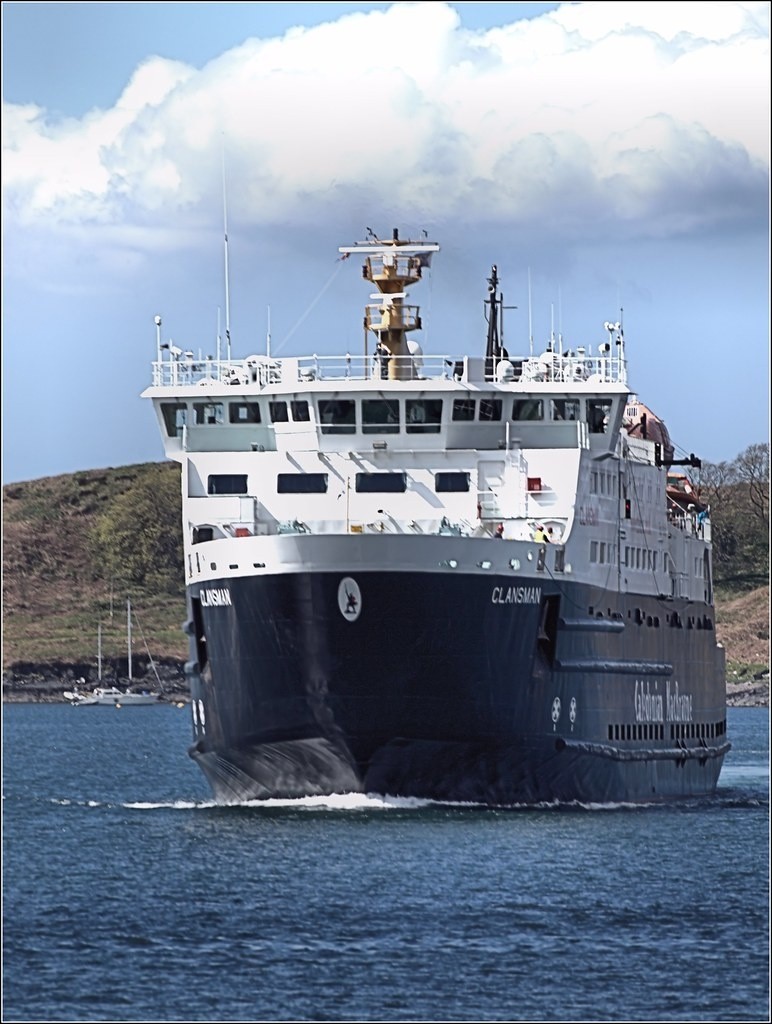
[144,228,733,806]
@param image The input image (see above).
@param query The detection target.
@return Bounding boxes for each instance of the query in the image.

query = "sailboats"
[63,597,164,707]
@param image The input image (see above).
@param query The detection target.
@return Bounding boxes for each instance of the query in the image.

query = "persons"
[410,400,425,433]
[698,505,709,531]
[495,525,503,539]
[589,403,606,433]
[373,341,391,380]
[534,526,551,544]
